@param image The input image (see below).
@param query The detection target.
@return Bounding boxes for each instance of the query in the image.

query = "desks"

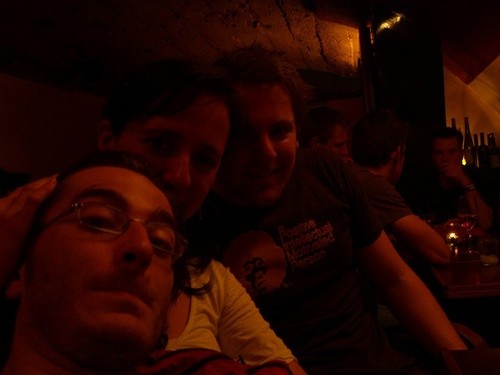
[421,249,500,320]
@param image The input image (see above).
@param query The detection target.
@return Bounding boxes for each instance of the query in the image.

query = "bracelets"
[464,184,474,192]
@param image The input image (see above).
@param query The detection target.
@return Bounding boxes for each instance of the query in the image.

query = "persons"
[0,55,309,375]
[0,150,294,375]
[185,43,500,375]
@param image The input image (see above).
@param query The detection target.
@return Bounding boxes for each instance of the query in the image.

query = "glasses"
[37,201,188,265]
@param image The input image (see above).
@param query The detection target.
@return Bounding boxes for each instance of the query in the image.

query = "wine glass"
[458,213,477,255]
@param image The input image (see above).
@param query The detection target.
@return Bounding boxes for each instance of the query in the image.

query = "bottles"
[452,119,456,128]
[478,132,488,169]
[487,132,496,169]
[464,117,475,166]
[474,134,479,168]
